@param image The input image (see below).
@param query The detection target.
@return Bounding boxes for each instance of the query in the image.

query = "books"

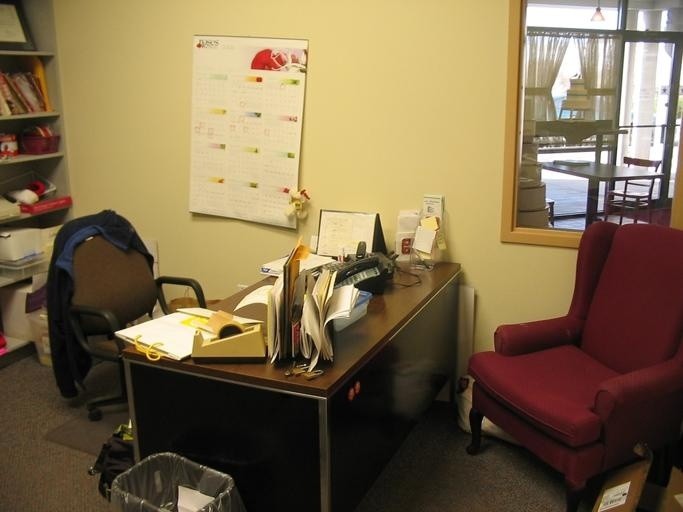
[110,242,357,370]
[0,57,51,120]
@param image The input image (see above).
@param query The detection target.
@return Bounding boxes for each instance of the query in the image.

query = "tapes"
[217,322,244,339]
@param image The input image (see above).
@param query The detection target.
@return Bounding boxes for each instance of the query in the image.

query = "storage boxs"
[592,459,682,512]
[25,306,52,367]
[0,272,48,340]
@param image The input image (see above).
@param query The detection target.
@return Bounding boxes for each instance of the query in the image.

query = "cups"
[408,234,435,271]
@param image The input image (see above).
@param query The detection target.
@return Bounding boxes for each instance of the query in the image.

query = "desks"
[119,251,462,512]
[536,160,665,230]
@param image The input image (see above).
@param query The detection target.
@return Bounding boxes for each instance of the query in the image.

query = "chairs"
[52,213,207,420]
[465,221,682,511]
[545,198,554,229]
[604,157,662,226]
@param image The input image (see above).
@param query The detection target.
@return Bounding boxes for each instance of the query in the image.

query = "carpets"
[43,403,130,457]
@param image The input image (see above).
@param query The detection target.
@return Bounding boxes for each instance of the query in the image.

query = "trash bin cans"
[111,452,234,512]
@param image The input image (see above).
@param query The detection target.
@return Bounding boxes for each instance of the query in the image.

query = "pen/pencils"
[337,248,346,263]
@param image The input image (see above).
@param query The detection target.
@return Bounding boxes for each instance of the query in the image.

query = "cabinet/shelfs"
[0,49,73,369]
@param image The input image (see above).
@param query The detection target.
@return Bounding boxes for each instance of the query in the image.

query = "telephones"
[336,256,387,295]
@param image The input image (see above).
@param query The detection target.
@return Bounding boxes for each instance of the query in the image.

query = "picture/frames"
[0,0,37,52]
